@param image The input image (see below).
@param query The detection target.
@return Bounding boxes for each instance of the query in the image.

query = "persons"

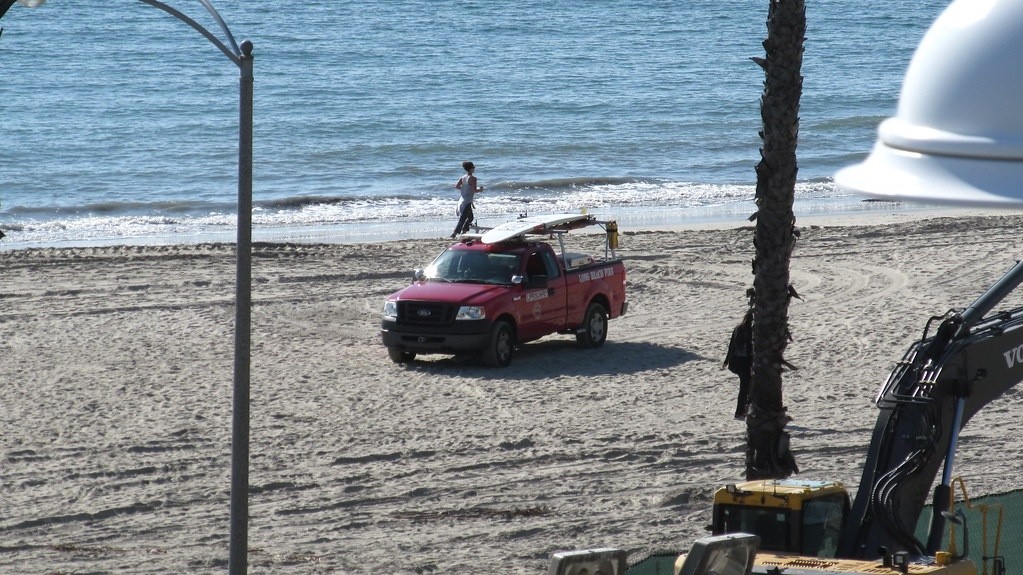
[450,160,483,237]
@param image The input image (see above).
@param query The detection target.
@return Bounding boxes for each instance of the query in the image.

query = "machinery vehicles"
[667,257,1023,575]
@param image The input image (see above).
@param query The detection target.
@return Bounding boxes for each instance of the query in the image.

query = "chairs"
[461,253,490,274]
[530,256,552,287]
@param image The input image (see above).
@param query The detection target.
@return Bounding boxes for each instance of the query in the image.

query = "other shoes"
[450,234,456,238]
[733,413,748,419]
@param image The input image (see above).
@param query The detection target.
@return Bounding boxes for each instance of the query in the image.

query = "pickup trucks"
[379,213,631,370]
